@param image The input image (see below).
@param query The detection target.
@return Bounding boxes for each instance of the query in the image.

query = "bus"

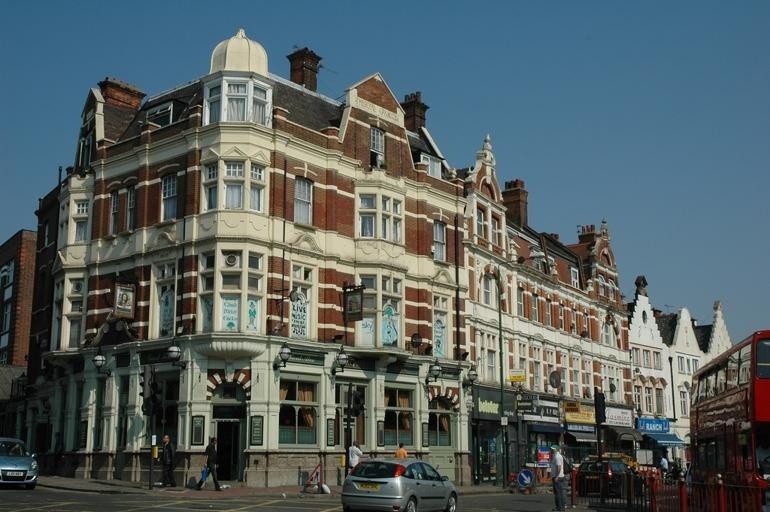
[688,327,770,512]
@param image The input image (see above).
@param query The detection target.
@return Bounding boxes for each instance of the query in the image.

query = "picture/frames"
[191,415,205,445]
[327,418,336,446]
[377,421,385,446]
[422,422,429,448]
[251,416,263,445]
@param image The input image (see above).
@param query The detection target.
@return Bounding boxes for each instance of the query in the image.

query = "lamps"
[273,341,291,370]
[332,343,348,375]
[425,359,442,384]
[462,366,478,388]
[167,337,187,370]
[17,371,29,386]
[93,346,111,377]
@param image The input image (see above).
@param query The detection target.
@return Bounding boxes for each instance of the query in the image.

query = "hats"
[550,445,559,451]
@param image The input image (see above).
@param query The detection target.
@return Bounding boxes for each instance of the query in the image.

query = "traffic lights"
[352,390,364,418]
[149,379,167,417]
[138,365,157,416]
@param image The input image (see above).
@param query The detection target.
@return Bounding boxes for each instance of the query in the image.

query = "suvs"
[576,459,647,498]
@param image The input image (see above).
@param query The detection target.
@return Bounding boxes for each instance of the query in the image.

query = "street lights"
[497,251,547,490]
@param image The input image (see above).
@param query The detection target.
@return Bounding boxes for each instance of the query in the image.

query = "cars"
[684,463,692,495]
[0,437,40,490]
[339,455,461,512]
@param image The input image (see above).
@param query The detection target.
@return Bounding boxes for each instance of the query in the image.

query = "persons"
[549,443,567,511]
[395,442,408,458]
[349,440,363,469]
[120,292,130,305]
[660,453,668,474]
[195,436,223,491]
[159,434,177,487]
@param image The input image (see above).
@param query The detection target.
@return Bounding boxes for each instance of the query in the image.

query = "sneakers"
[552,506,562,511]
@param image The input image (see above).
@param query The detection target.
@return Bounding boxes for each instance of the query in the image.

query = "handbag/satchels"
[202,467,206,481]
